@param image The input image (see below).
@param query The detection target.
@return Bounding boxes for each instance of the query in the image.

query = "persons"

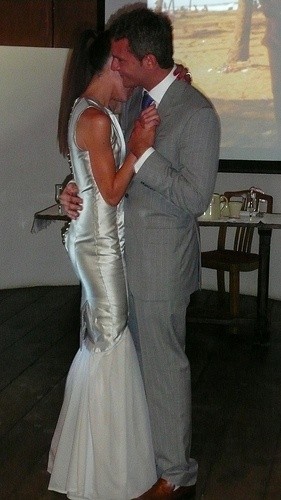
[48,24,192,500]
[57,1,221,500]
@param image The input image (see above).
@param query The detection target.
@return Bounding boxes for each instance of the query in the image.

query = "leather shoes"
[130,478,194,500]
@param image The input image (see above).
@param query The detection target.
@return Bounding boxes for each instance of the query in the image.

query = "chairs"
[200,191,274,320]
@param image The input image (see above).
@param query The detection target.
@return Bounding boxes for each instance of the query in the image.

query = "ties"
[141,91,154,110]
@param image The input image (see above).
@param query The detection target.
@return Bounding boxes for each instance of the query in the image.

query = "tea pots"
[204,194,228,220]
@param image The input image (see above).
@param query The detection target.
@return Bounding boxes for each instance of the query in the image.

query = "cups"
[217,201,243,219]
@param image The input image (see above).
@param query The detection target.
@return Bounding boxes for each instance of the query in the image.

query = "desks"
[34,200,281,353]
